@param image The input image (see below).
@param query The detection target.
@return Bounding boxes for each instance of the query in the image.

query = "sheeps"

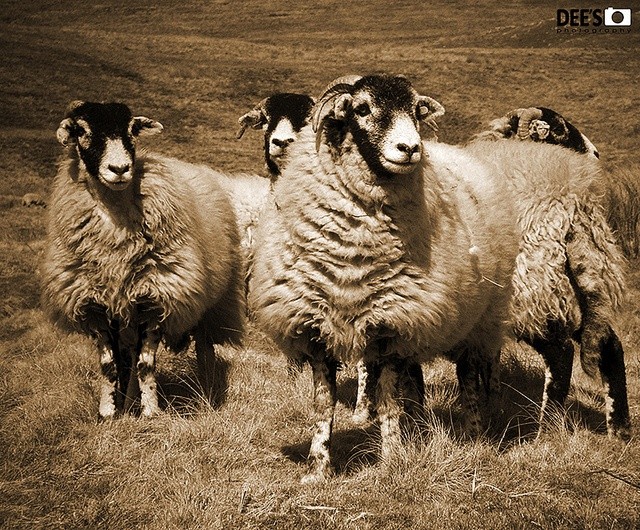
[47,100,273,424]
[249,75,518,483]
[191,166,273,398]
[460,105,631,443]
[236,90,315,390]
